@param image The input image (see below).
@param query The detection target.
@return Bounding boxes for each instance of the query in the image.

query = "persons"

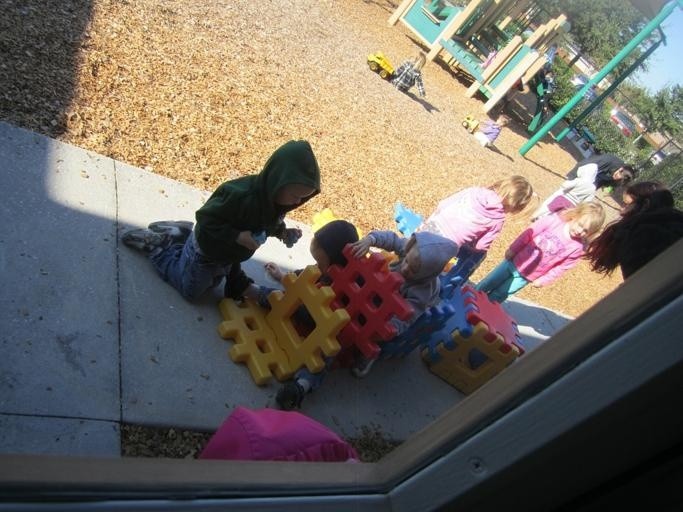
[474,202,605,304]
[390,50,430,100]
[531,69,556,128]
[224,220,360,412]
[348,230,459,379]
[123,140,320,301]
[473,114,512,148]
[529,163,598,222]
[480,48,498,67]
[416,175,533,286]
[582,181,683,279]
[566,154,639,191]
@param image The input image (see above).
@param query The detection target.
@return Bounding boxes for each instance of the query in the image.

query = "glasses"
[621,201,637,208]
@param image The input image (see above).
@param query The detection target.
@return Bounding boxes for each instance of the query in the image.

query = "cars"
[609,108,637,139]
[569,72,597,103]
[648,149,667,166]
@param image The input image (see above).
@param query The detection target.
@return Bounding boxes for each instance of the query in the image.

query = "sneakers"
[149,220,193,243]
[122,229,168,252]
[353,347,380,377]
